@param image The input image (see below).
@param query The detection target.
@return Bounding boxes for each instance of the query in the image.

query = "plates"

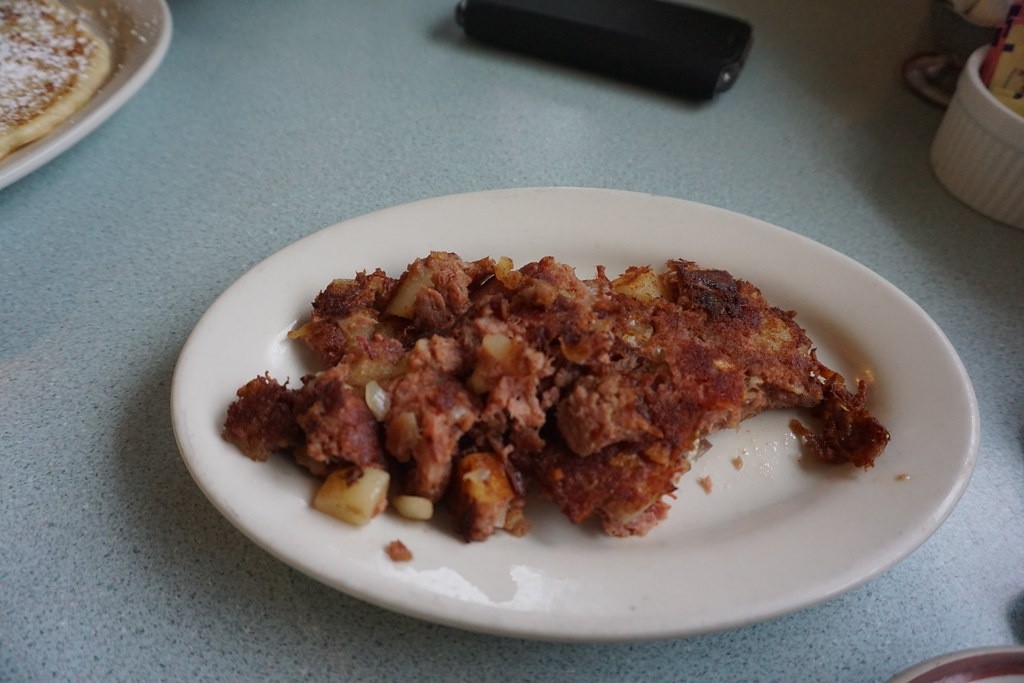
[169,187,982,643]
[0,0,174,192]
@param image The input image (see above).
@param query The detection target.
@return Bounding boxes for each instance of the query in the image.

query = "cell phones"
[455,0,753,100]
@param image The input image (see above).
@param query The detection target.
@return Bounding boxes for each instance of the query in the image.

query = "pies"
[0,0,113,164]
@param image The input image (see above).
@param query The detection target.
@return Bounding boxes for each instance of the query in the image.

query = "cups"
[930,44,1024,230]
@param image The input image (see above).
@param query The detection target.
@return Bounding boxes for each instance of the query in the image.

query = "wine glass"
[902,0,1003,108]
